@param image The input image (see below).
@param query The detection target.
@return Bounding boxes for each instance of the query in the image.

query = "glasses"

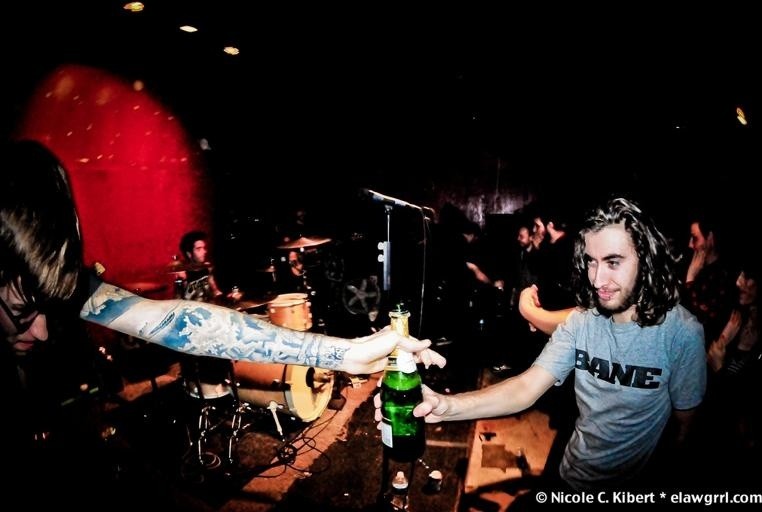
[0,294,55,333]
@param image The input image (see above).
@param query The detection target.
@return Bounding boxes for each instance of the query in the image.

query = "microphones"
[358,187,422,211]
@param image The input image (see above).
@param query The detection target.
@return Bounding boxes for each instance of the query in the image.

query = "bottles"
[392,472,410,511]
[382,304,427,462]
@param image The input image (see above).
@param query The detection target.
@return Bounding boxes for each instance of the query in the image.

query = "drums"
[267,293,313,331]
[184,381,232,400]
[227,360,335,422]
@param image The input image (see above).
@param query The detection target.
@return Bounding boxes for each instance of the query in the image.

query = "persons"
[0,138,448,512]
[373,198,710,512]
[95,196,762,472]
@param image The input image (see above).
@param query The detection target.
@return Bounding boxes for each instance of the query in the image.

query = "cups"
[427,470,443,492]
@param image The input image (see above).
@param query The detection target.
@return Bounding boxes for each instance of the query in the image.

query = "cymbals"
[154,259,211,273]
[228,301,278,310]
[276,237,332,249]
